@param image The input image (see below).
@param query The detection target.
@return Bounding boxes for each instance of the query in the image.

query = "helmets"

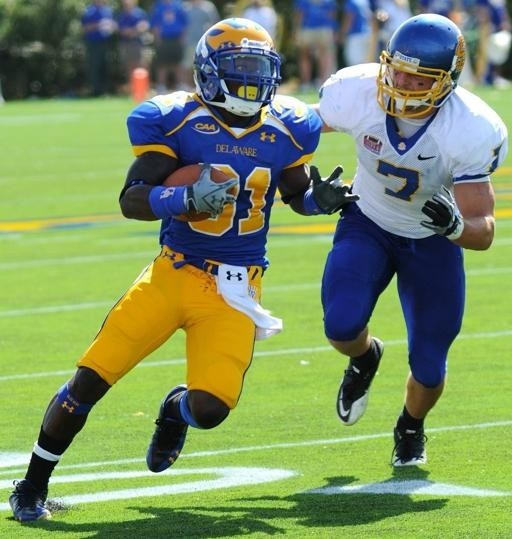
[383,11,467,108]
[192,17,281,101]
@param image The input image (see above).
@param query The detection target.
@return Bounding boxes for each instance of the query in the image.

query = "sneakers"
[335,336,385,427]
[145,384,189,473]
[8,479,52,522]
[390,412,427,469]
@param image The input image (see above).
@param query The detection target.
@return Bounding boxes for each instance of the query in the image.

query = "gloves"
[311,163,360,216]
[185,162,240,217]
[420,182,465,242]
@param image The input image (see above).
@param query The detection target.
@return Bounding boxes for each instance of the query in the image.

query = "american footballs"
[159,165,235,222]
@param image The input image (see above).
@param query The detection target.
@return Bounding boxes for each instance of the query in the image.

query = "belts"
[182,255,250,277]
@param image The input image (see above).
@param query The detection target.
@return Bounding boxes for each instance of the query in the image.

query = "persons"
[303,12,509,468]
[80,0,278,98]
[8,16,360,525]
[294,0,512,87]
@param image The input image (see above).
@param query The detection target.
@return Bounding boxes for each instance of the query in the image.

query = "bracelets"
[303,186,329,215]
[149,184,190,220]
[446,217,464,242]
